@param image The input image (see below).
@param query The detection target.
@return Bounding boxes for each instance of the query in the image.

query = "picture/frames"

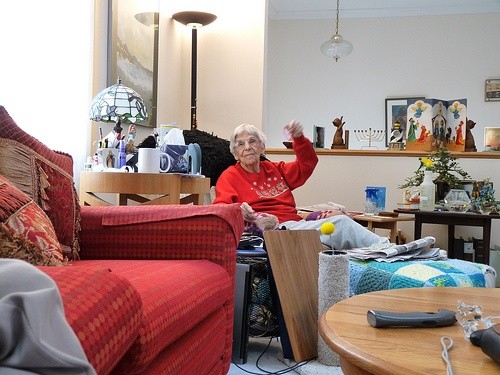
[385,96,426,147]
[484,126,500,153]
[107,0,160,129]
[485,79,500,102]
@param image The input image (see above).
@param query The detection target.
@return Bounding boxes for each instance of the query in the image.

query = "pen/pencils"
[115,135,125,149]
[97,127,109,148]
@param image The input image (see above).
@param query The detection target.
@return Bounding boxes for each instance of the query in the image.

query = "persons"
[213,118,390,250]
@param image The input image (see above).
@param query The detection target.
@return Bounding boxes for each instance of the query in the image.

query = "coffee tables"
[317,287,500,375]
[393,202,500,267]
[348,211,414,244]
[348,257,497,298]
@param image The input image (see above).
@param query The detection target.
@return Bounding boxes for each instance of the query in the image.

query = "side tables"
[79,170,210,207]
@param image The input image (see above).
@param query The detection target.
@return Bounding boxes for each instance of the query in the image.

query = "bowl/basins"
[283,142,313,149]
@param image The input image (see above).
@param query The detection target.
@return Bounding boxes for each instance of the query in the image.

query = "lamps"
[171,11,218,130]
[320,0,353,61]
[135,12,159,128]
[88,76,149,168]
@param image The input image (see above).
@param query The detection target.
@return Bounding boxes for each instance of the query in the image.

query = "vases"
[479,205,496,215]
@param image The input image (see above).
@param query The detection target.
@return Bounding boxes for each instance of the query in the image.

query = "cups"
[361,198,376,216]
[137,148,171,174]
[97,148,118,169]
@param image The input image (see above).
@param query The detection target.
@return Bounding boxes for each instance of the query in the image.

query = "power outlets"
[464,243,473,253]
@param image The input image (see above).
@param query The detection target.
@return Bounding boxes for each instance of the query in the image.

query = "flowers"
[398,145,476,191]
[432,173,452,205]
[468,176,500,208]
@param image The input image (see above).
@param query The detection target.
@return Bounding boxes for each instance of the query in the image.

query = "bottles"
[444,189,472,213]
[419,171,435,212]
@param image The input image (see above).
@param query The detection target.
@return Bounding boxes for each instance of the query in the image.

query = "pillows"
[0,174,69,267]
[0,105,81,261]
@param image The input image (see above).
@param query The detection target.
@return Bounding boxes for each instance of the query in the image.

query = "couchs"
[0,104,245,375]
[164,128,333,360]
